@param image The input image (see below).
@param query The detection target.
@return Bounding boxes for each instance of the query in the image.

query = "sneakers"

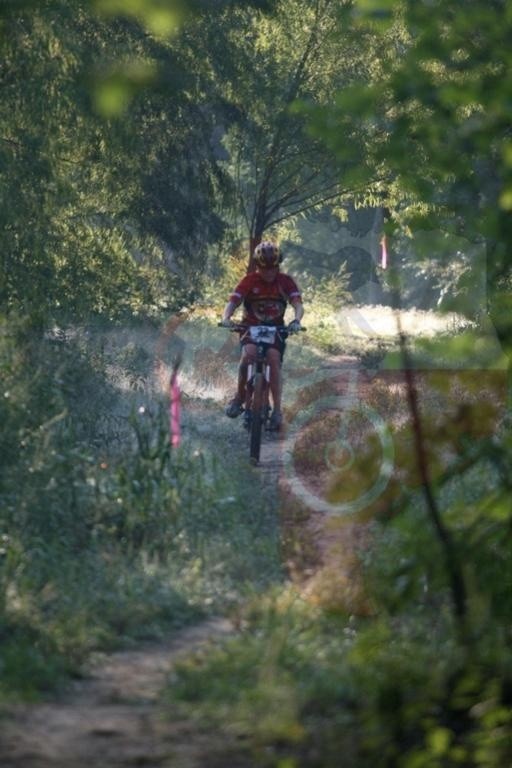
[227,398,240,417]
[271,413,283,429]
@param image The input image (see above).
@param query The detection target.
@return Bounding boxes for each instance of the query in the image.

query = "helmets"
[252,241,281,268]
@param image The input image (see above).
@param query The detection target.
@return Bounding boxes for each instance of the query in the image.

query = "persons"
[222,239,305,433]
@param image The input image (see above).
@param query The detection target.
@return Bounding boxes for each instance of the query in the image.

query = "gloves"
[288,319,302,335]
[221,318,233,327]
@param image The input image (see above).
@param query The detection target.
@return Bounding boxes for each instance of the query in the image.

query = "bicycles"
[214,320,309,466]
